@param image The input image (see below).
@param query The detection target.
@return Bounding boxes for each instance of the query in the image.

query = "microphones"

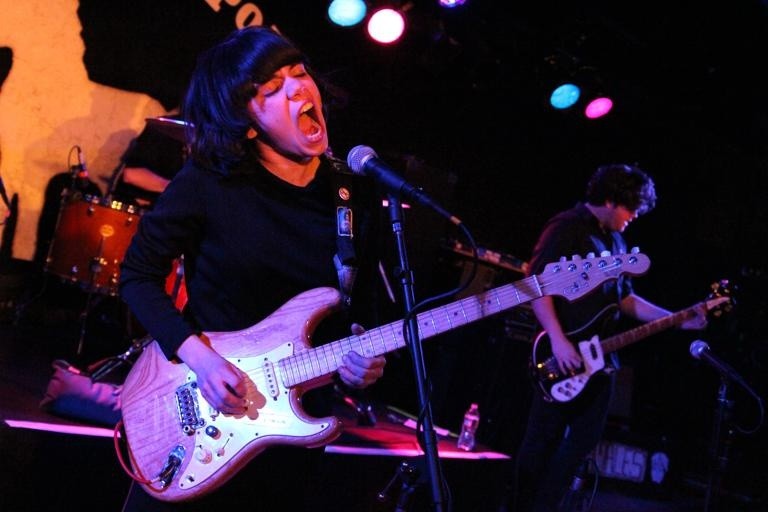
[77,150,89,187]
[347,144,462,228]
[689,339,764,403]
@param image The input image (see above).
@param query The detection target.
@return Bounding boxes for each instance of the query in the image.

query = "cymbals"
[144,115,195,136]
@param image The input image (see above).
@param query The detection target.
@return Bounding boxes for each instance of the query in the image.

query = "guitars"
[119,246,651,503]
[532,281,737,404]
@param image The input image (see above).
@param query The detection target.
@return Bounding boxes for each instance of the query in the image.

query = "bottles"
[457,403,480,451]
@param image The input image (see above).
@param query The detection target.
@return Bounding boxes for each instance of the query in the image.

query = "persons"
[118,28,390,510]
[509,165,708,511]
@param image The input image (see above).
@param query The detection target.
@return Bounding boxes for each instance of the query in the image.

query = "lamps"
[537,52,615,121]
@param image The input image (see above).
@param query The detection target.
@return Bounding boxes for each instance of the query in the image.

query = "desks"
[0,370,511,512]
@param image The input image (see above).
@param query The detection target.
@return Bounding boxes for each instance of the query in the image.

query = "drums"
[43,190,190,315]
